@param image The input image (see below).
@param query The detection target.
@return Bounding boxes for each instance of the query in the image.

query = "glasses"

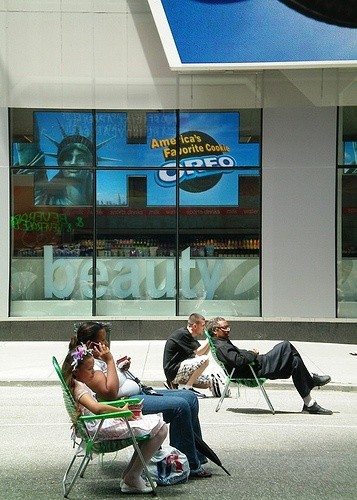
[217,325,230,331]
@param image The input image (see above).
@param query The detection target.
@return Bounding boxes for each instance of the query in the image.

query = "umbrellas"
[136,377,231,476]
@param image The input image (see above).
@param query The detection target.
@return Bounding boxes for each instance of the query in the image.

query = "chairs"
[52,358,160,497]
[205,329,276,414]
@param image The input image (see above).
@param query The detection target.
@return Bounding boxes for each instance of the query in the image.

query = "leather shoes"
[311,372,331,387]
[302,401,333,415]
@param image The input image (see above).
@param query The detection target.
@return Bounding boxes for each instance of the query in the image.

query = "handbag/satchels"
[210,373,232,397]
[143,445,190,486]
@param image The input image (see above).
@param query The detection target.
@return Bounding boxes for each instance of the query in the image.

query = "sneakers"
[183,387,206,398]
[163,380,178,390]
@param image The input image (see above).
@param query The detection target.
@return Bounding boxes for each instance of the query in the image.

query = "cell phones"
[88,341,100,352]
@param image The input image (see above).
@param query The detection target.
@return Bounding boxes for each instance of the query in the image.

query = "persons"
[69,322,213,477]
[206,318,333,415]
[61,344,167,493]
[163,313,210,398]
[42,119,122,205]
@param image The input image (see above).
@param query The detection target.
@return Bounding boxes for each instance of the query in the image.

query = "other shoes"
[120,478,157,493]
[190,466,212,478]
